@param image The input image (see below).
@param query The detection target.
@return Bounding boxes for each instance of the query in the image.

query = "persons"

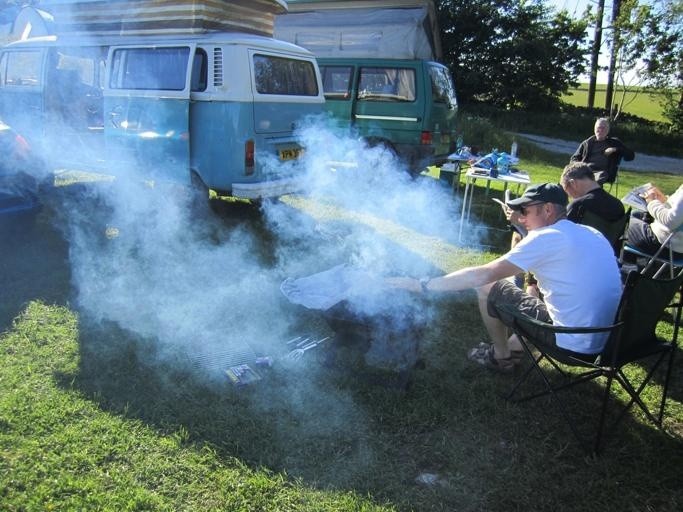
[568,117,634,188]
[502,161,624,290]
[381,182,624,378]
[621,181,683,260]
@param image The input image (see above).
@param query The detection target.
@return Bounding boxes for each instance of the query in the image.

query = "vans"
[314,58,459,171]
[0,36,325,210]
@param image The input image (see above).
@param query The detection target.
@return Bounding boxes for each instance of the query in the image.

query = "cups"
[490,169,498,178]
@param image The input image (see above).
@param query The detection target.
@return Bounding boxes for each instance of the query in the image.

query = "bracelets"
[420,276,430,292]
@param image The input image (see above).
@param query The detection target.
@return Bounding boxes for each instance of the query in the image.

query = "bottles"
[496,151,509,175]
[511,141,518,157]
[455,135,464,156]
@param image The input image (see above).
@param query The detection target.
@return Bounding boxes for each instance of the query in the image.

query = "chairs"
[458,162,531,251]
[497,270,683,467]
[620,225,683,282]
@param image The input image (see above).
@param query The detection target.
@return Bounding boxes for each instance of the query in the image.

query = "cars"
[0,119,56,224]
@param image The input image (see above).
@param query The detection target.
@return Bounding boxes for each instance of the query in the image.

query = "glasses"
[520,200,549,216]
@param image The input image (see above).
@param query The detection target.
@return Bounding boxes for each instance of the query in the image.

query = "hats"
[507,181,569,206]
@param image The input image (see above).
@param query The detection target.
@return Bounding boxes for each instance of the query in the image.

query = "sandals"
[466,340,527,378]
